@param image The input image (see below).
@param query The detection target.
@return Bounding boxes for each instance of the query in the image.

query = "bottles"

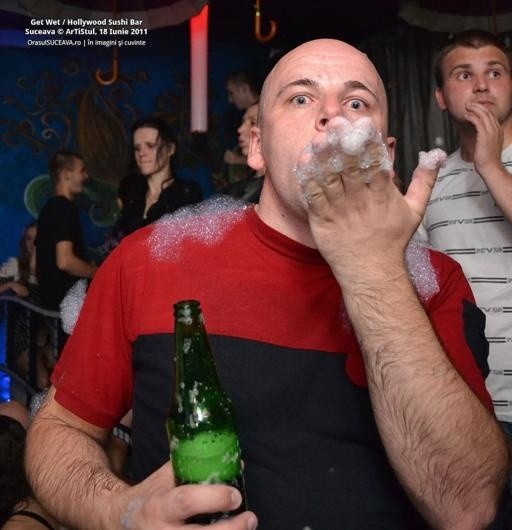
[170,302,248,525]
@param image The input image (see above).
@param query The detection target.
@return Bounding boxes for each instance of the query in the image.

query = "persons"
[0,29,512,530]
